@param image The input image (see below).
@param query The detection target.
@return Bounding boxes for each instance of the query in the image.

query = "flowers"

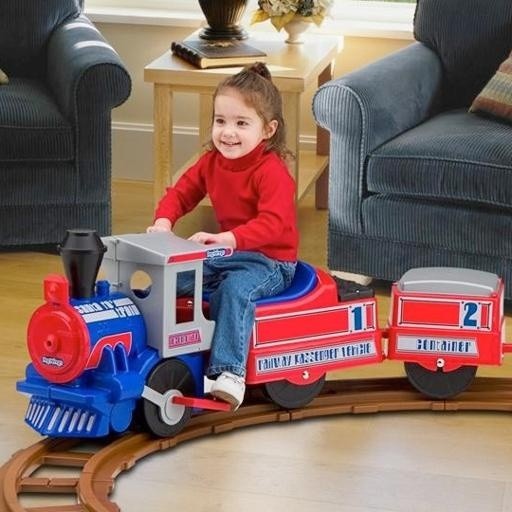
[250,0,331,32]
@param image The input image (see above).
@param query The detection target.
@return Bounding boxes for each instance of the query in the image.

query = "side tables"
[143,26,345,211]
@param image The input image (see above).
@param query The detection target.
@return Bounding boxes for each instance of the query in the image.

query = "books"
[171,38,268,69]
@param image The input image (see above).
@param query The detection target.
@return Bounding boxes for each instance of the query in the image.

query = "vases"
[199,1,248,42]
[281,17,310,43]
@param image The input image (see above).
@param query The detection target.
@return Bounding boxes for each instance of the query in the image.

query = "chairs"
[1,1,131,254]
[312,2,511,300]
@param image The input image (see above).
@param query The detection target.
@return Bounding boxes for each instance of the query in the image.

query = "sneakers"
[209,371,246,414]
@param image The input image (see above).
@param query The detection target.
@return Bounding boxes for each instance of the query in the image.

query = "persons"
[147,63,299,411]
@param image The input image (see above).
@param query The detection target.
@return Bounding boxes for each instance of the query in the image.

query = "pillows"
[468,52,510,119]
